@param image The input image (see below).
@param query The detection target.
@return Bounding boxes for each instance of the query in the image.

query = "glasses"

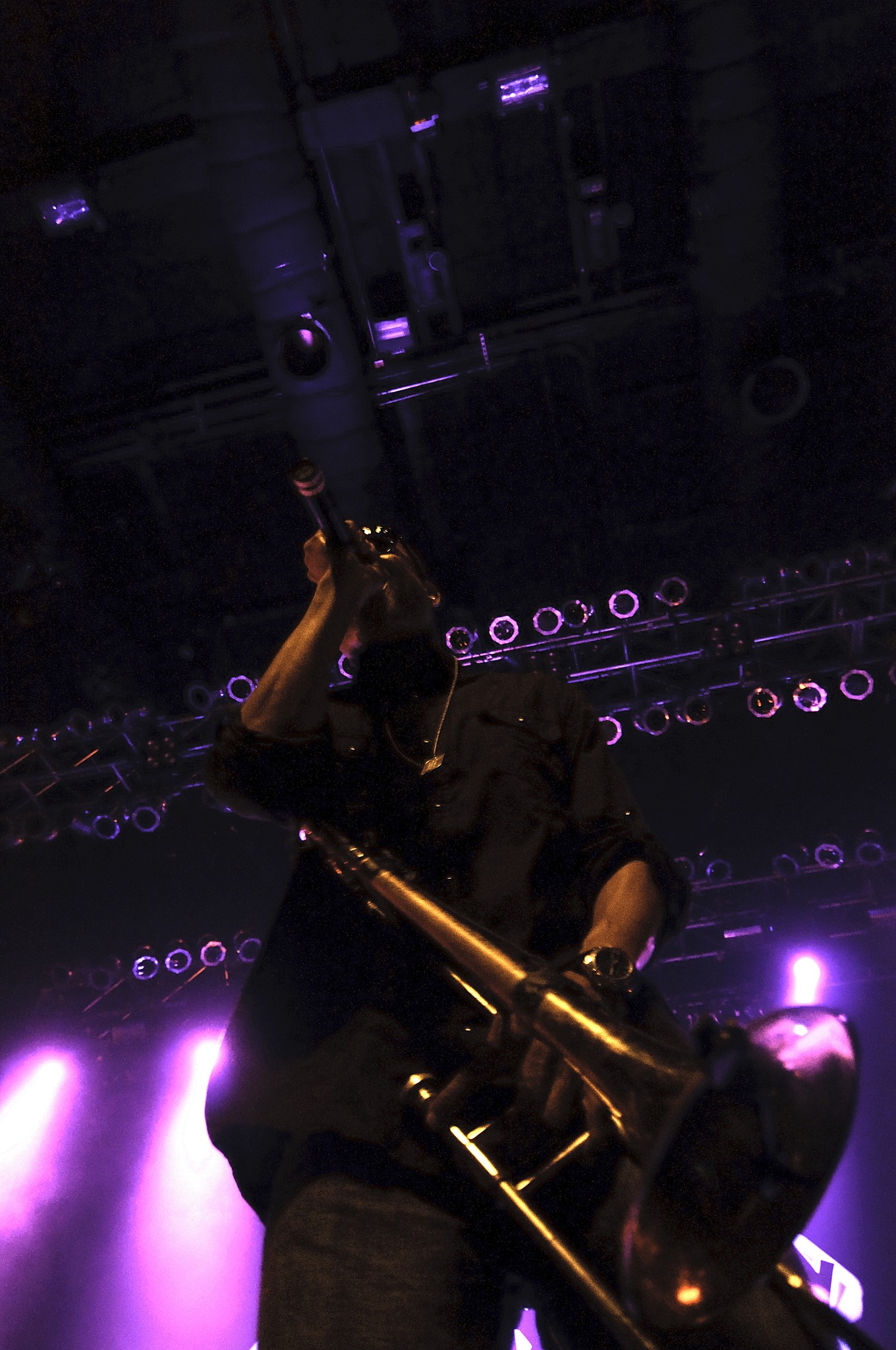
[358,525,426,580]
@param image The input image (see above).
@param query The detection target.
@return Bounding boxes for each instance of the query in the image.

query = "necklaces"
[384,655,458,777]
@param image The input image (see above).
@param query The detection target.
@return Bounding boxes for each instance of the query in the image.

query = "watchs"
[559,946,655,1015]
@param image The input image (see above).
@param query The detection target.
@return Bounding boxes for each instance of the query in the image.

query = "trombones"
[292,818,863,1350]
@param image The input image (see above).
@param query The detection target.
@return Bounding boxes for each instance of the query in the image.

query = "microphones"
[294,459,359,553]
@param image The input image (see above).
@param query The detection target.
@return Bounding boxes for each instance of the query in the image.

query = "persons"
[204,519,821,1350]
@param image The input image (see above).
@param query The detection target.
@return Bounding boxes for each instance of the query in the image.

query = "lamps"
[840,665,875,701]
[28,167,110,244]
[672,696,713,724]
[792,673,831,713]
[337,652,357,680]
[748,682,784,720]
[369,312,420,363]
[443,572,687,657]
[729,538,895,596]
[673,830,888,887]
[597,715,624,747]
[632,704,672,738]
[0,672,262,850]
[27,927,269,1047]
